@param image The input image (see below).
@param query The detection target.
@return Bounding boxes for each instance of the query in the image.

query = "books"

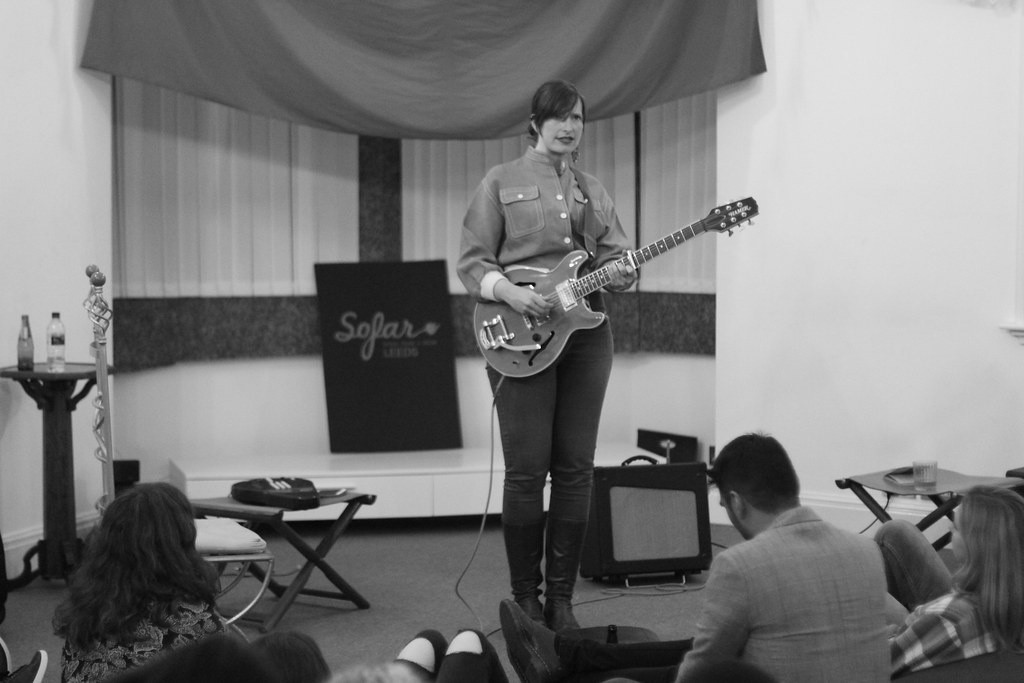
[883,466,930,487]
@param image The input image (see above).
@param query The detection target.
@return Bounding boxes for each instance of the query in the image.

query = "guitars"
[474,196,760,379]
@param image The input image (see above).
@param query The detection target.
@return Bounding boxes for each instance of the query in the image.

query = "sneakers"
[515,596,546,632]
[542,599,578,636]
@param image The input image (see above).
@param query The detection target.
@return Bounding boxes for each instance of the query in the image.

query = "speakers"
[579,463,713,582]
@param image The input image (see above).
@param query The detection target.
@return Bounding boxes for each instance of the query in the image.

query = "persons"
[0,530,780,683]
[875,484,1024,683]
[51,480,231,683]
[500,430,892,682]
[456,79,640,636]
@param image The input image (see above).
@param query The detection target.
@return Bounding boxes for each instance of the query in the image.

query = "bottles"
[47,310,67,372]
[17,314,35,370]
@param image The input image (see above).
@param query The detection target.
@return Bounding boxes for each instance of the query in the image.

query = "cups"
[911,459,938,492]
